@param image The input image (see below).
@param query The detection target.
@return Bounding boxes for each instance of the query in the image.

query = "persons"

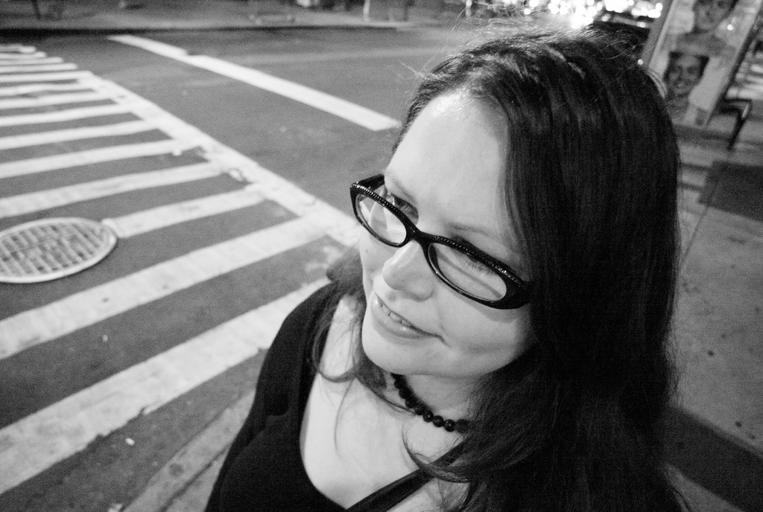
[201,32,691,512]
[663,1,738,71]
[657,51,710,130]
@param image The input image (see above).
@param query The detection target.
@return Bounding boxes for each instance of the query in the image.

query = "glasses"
[350,175,533,310]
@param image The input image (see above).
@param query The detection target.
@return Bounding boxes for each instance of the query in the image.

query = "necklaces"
[390,372,474,436]
